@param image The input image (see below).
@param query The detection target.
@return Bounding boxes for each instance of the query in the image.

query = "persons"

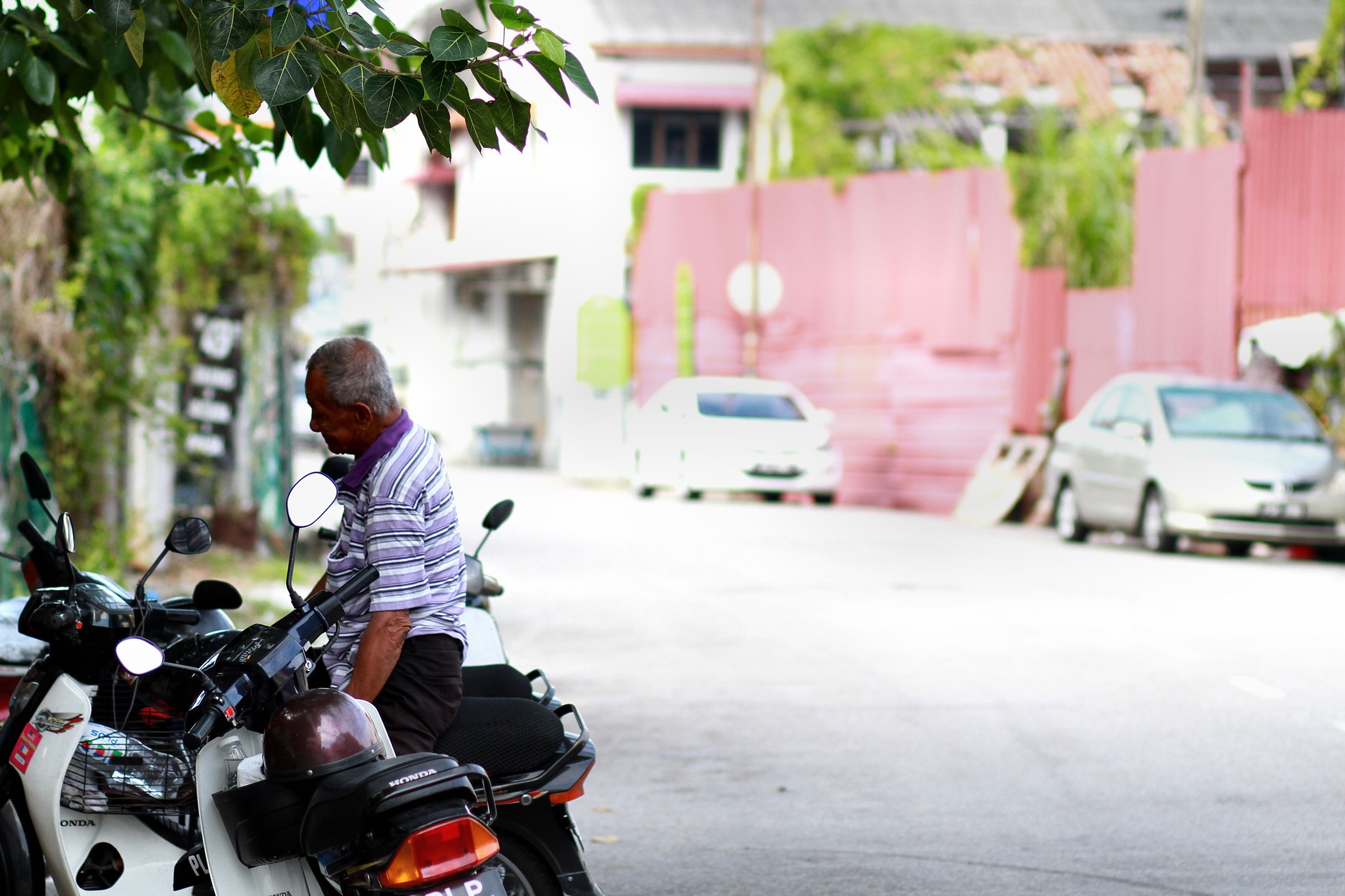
[303,337,468,755]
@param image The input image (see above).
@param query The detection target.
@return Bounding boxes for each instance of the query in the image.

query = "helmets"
[261,688,384,781]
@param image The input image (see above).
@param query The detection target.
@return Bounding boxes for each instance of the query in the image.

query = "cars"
[625,376,841,509]
[1035,370,1344,563]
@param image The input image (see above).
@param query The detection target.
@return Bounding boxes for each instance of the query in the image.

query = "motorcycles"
[0,509,600,895]
[1,448,557,703]
[113,472,540,895]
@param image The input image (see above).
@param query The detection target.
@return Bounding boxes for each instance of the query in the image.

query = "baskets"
[60,706,207,816]
